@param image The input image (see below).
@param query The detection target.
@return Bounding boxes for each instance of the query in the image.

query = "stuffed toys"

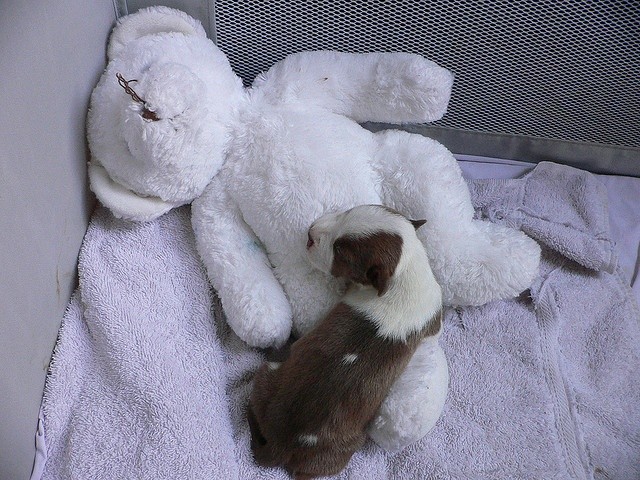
[84,6,542,451]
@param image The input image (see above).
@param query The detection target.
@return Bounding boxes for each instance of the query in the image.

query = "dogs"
[244,202,445,480]
